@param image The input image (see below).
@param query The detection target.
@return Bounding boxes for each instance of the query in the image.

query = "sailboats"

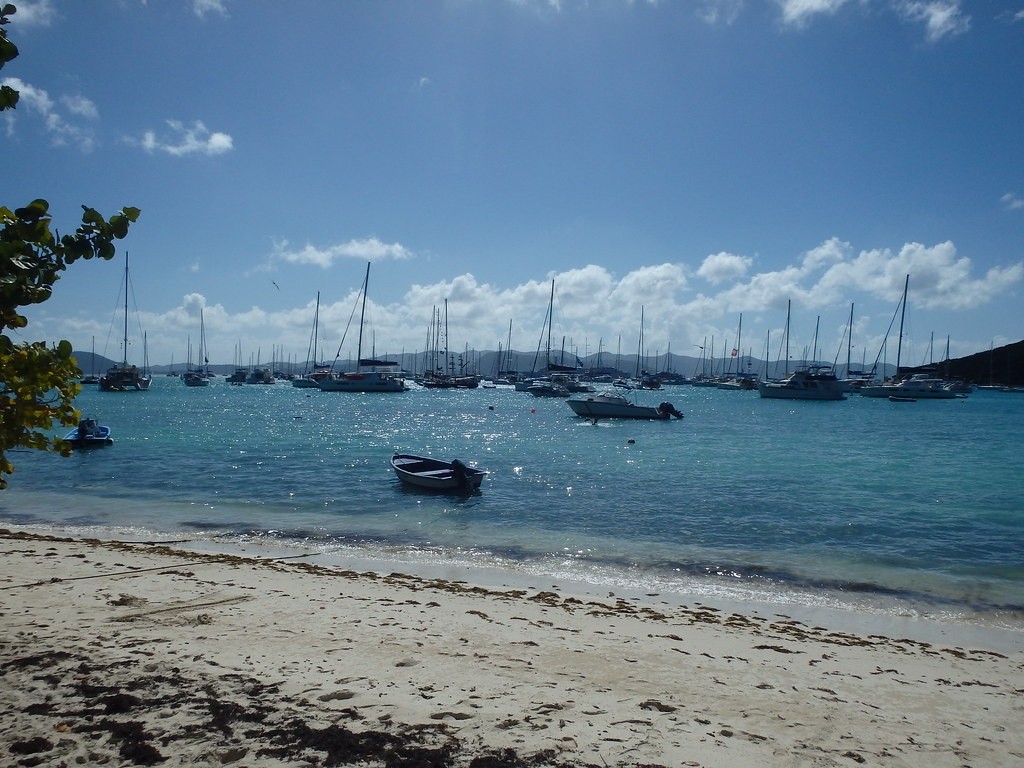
[165,307,216,386]
[226,261,1024,400]
[81,250,153,392]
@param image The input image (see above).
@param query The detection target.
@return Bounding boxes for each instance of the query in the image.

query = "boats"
[564,383,685,420]
[389,450,486,494]
[61,414,111,448]
[889,395,917,402]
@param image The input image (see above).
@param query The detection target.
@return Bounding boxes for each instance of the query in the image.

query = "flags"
[731,348,737,356]
[577,356,583,367]
